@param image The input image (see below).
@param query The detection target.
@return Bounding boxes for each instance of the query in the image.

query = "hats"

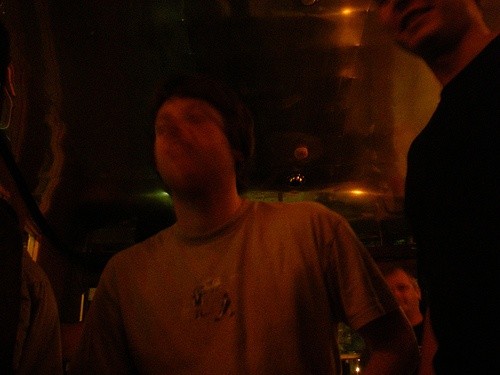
[153,64,255,164]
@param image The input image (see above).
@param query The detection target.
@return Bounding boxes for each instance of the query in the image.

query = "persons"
[0,18,64,375]
[67,86,418,375]
[371,0,500,375]
[360,264,425,375]
